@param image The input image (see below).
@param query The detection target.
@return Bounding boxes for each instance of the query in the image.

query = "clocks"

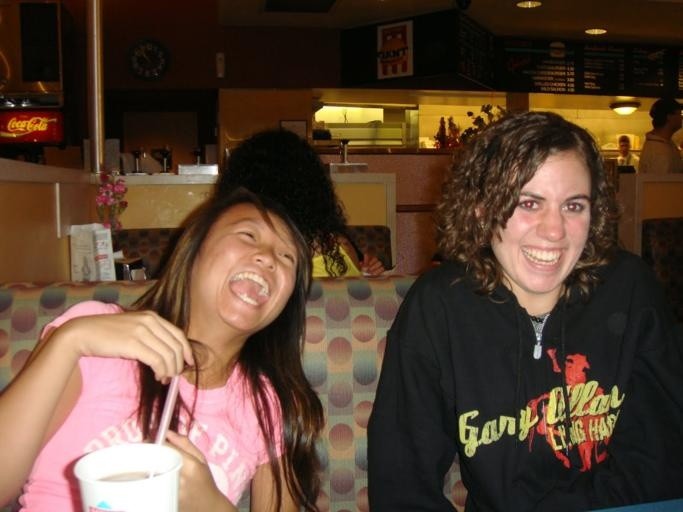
[125,38,169,81]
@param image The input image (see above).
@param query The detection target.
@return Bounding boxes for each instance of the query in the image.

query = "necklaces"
[526,313,547,326]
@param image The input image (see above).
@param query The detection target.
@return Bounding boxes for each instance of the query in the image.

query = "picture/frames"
[376,20,413,80]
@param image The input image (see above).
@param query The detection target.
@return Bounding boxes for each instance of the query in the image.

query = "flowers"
[93,161,128,240]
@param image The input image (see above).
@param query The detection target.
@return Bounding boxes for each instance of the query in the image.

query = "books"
[67,221,119,284]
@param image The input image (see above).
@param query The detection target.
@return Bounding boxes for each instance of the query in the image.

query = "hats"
[649,97,683,117]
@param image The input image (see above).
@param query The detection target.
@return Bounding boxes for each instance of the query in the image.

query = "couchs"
[640,217,682,323]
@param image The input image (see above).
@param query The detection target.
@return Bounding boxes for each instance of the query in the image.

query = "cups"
[72,442,183,512]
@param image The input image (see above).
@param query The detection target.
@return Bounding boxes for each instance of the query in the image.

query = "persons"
[207,124,390,281]
[613,132,639,175]
[0,185,329,511]
[363,109,681,511]
[638,94,682,321]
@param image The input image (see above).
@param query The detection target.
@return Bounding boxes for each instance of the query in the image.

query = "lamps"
[609,103,641,116]
